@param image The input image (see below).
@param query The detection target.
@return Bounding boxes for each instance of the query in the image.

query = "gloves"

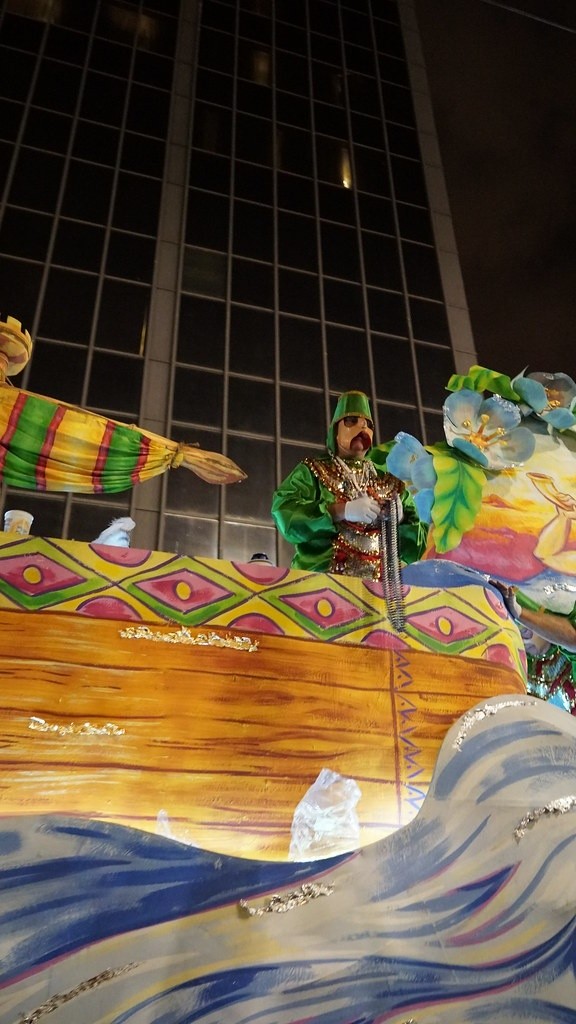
[345,496,380,524]
[381,494,403,523]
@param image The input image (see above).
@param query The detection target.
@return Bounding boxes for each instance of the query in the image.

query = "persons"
[488,580,576,654]
[270,391,430,582]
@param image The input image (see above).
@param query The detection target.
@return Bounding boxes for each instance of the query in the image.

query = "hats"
[326,391,374,458]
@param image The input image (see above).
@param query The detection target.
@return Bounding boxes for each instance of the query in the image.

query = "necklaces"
[334,455,371,498]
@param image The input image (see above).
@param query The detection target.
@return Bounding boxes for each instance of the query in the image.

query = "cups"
[4,510,34,534]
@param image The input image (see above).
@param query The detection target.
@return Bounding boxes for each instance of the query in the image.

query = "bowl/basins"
[248,559,272,566]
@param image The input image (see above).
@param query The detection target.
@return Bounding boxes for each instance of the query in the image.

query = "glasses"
[344,416,374,431]
